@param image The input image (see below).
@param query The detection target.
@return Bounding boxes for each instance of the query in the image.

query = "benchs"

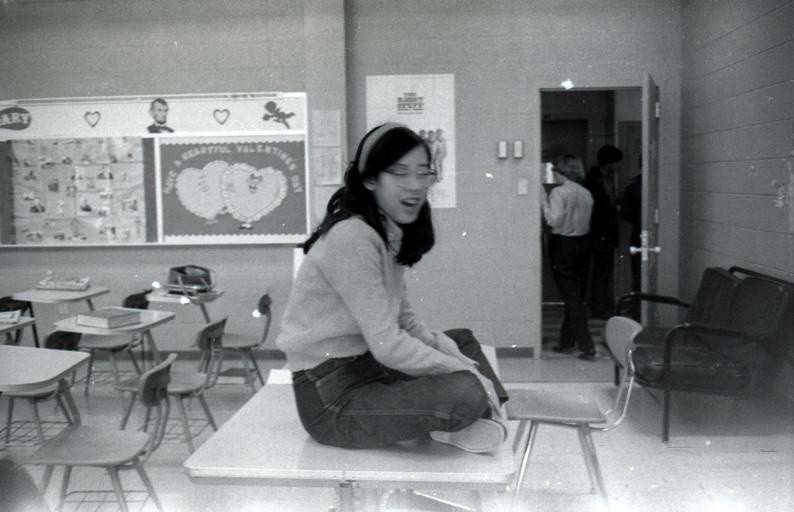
[615,266,794,442]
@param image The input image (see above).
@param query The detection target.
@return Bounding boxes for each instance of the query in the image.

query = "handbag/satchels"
[168,265,211,295]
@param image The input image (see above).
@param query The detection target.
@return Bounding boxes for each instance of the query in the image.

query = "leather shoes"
[553,343,596,362]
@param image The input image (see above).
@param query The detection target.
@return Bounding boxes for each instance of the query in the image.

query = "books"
[35,276,89,291]
[75,308,142,328]
[0,309,21,323]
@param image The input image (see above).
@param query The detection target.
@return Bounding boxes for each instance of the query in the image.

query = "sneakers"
[428,416,507,454]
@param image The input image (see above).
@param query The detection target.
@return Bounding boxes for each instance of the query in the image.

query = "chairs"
[191,293,273,395]
[114,317,228,454]
[505,316,642,506]
[17,353,179,512]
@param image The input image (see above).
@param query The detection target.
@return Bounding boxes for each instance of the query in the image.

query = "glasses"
[385,166,437,188]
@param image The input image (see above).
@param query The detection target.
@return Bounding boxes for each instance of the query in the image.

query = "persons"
[541,153,595,361]
[419,128,446,182]
[147,98,173,133]
[29,196,44,212]
[276,122,509,454]
[97,163,113,179]
[81,196,92,211]
[581,144,641,321]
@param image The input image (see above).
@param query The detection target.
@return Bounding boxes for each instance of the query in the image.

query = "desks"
[144,289,225,324]
[0,316,36,345]
[55,306,176,412]
[182,343,517,512]
[10,286,110,310]
[0,344,91,445]
